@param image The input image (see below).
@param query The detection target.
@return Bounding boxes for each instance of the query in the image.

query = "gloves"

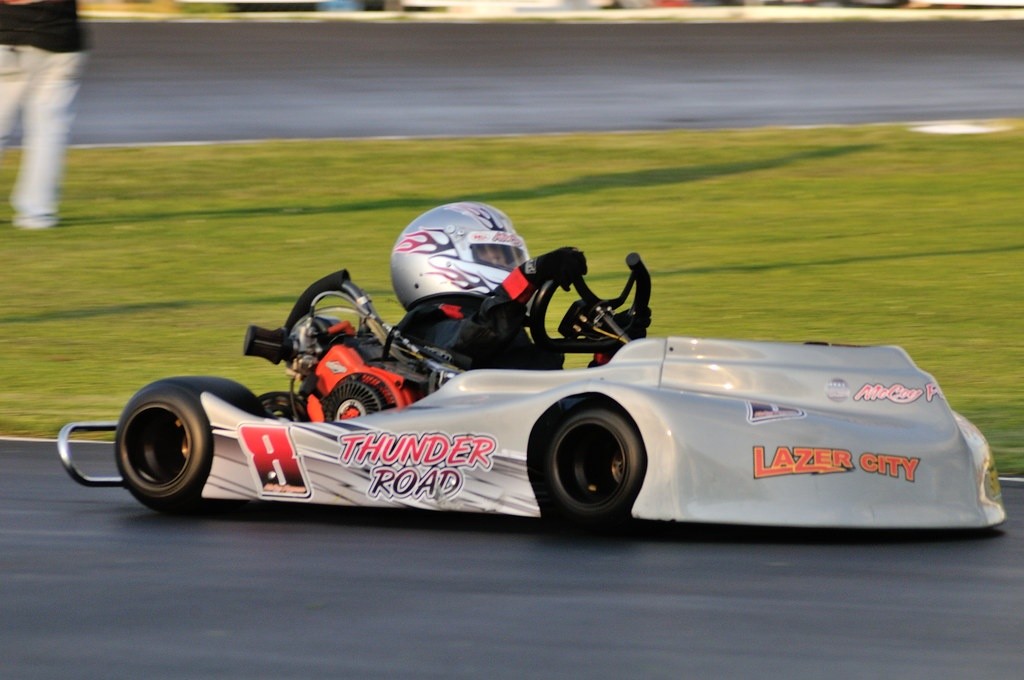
[603,305,651,343]
[518,246,588,292]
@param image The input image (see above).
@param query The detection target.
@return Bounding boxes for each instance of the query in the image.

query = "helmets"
[390,201,531,311]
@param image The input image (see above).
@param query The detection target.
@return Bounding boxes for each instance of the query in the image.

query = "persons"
[390,200,653,371]
[0,0,94,230]
